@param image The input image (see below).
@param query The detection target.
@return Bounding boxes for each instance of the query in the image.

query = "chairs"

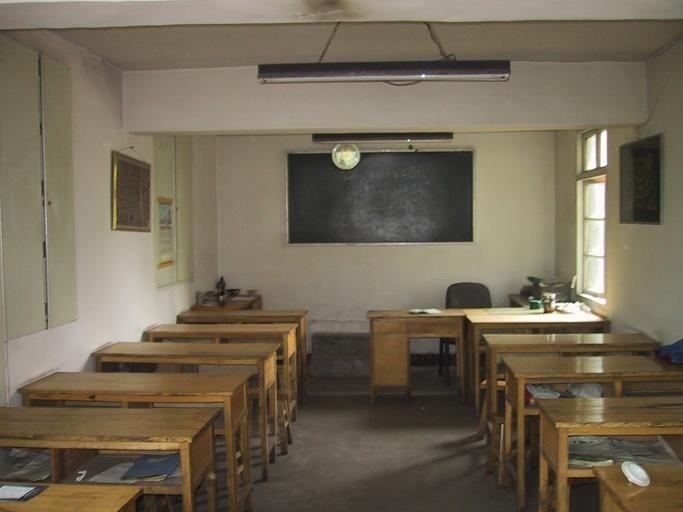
[438,283,494,384]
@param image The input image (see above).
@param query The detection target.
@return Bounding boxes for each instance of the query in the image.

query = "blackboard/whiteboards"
[284,147,476,246]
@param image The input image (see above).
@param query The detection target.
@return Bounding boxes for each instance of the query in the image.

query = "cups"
[218,296,225,305]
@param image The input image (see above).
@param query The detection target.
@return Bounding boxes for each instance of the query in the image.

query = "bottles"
[217,277,225,296]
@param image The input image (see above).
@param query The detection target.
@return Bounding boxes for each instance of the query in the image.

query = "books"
[120,453,180,481]
[407,308,443,314]
[568,453,613,468]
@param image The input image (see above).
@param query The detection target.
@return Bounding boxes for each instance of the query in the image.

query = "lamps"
[312,131,455,141]
[258,20,510,84]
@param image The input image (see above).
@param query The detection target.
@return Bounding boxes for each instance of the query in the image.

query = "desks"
[90,340,282,483]
[462,306,610,416]
[0,405,223,512]
[535,395,683,512]
[591,463,683,512]
[178,309,308,408]
[502,354,683,512]
[17,370,253,511]
[145,322,298,422]
[192,294,264,313]
[0,481,147,512]
[482,334,663,412]
[368,309,513,403]
